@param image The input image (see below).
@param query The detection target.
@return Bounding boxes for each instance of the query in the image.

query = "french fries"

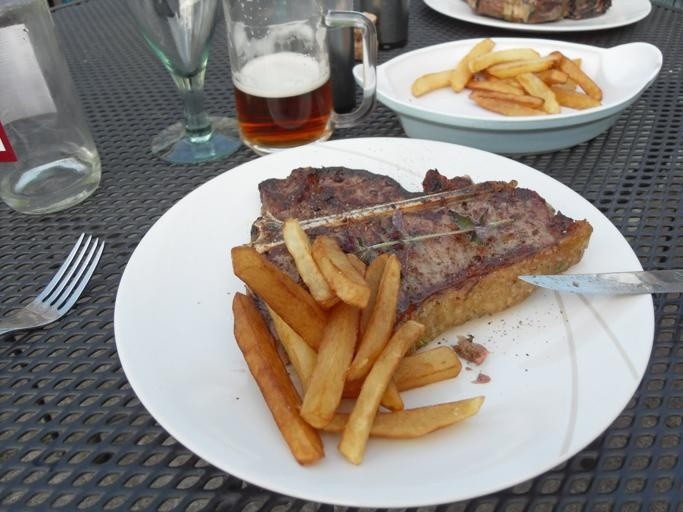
[231,218,485,466]
[413,38,603,116]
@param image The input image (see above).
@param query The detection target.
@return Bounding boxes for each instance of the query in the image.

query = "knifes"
[520,269,683,297]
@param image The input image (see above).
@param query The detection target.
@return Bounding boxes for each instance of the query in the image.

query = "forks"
[0,232,105,337]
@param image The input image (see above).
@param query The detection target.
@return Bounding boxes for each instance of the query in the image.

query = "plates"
[351,36,664,157]
[424,1,651,32]
[113,136,655,508]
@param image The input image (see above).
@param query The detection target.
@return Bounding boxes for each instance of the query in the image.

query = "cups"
[226,0,376,160]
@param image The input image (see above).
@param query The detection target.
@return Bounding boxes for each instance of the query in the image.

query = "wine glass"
[129,0,242,162]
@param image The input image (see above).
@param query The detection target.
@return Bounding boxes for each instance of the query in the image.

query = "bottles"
[1,2,101,221]
